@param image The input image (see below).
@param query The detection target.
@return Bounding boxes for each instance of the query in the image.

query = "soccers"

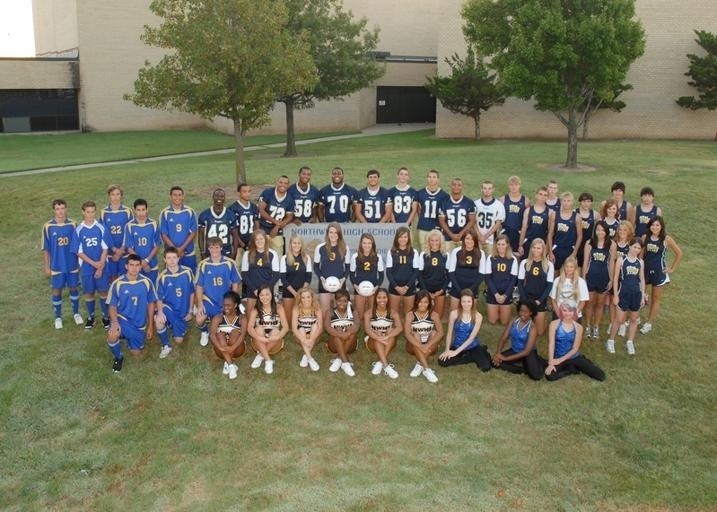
[325,276,339,292]
[358,281,374,297]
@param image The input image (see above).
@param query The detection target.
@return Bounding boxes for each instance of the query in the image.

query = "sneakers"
[300,354,320,371]
[55,318,62,329]
[372,361,398,379]
[410,364,438,383]
[329,358,355,376]
[223,362,238,379]
[584,317,651,355]
[112,360,122,372]
[74,314,110,328]
[251,355,273,374]
[159,345,172,359]
[200,331,209,346]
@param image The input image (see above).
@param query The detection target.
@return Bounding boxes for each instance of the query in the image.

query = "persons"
[41,165,682,383]
